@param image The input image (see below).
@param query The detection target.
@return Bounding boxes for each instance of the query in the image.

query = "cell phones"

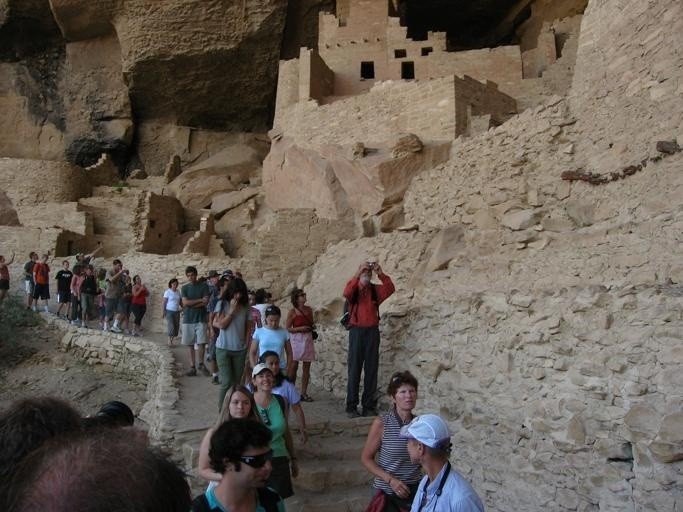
[126,270,129,274]
[97,242,100,244]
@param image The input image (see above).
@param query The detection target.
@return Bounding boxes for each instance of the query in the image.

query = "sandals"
[301,392,313,402]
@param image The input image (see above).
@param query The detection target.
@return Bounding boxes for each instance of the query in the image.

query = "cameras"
[368,261,376,270]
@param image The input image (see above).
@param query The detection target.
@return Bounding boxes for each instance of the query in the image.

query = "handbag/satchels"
[366,488,410,512]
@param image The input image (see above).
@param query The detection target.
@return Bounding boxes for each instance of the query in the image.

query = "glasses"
[239,448,272,469]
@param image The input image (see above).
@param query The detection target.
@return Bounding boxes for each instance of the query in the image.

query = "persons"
[342,260,396,419]
[360,370,426,512]
[397,412,485,512]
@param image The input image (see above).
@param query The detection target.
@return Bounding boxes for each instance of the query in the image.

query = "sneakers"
[184,368,196,376]
[212,376,218,385]
[110,326,123,333]
[198,365,210,376]
[362,407,377,415]
[346,409,361,418]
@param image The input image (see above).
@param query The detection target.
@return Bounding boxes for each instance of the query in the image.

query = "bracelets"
[388,476,393,487]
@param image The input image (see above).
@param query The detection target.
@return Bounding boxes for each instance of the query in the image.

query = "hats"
[222,270,235,279]
[252,289,272,298]
[252,363,274,379]
[400,413,451,449]
[207,270,221,280]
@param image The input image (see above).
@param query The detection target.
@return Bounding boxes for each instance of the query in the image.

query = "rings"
[398,490,401,493]
